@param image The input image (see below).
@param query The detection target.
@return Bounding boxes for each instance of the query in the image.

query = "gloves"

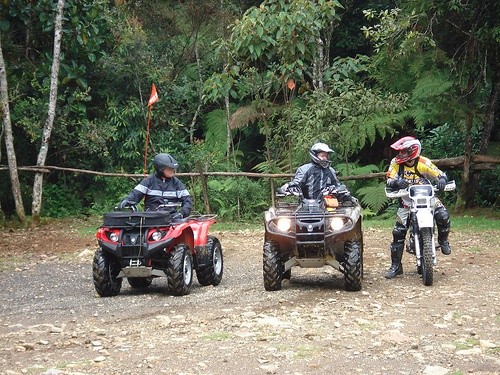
[118,199,137,210]
[287,186,303,197]
[351,196,360,206]
[170,213,183,220]
[437,176,447,190]
[390,178,409,189]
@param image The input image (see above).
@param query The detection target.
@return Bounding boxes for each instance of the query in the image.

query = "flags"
[147,83,159,107]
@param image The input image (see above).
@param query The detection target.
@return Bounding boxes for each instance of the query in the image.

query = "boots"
[437,231,451,255]
[385,242,404,278]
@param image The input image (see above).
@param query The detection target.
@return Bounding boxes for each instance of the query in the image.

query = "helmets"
[309,142,335,168]
[390,136,421,164]
[153,152,180,179]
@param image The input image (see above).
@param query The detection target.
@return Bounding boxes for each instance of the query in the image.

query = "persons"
[119,152,192,219]
[280,143,359,205]
[385,136,451,279]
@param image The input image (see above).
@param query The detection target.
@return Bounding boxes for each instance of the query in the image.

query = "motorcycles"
[384,175,456,287]
[93,197,223,298]
[262,184,365,291]
